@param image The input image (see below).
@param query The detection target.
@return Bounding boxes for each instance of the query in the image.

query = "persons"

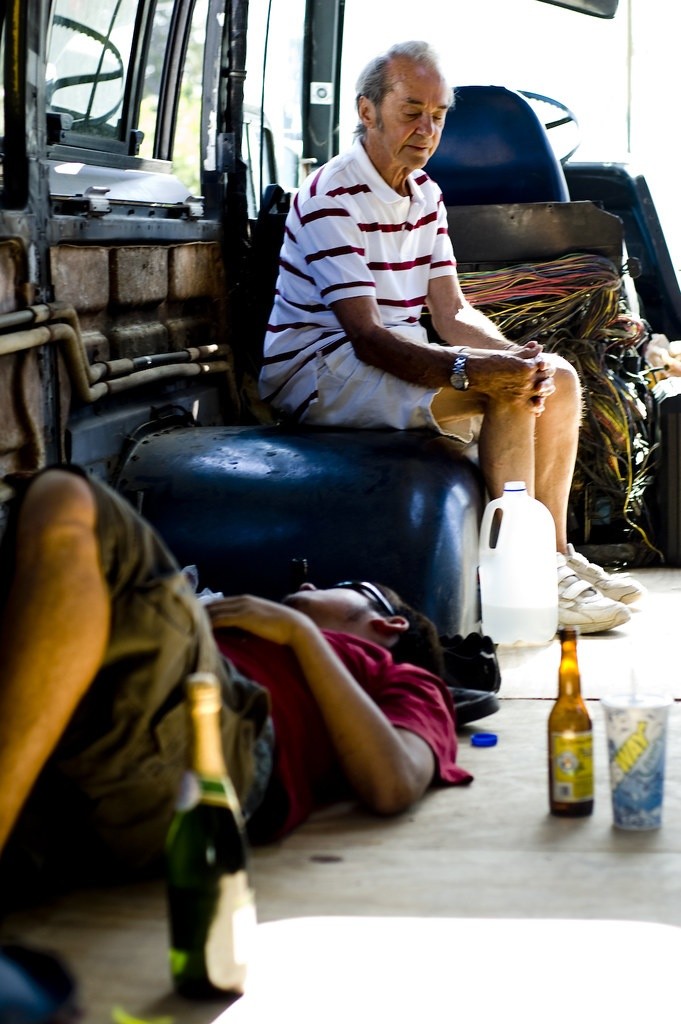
[256,36,641,631]
[0,462,473,868]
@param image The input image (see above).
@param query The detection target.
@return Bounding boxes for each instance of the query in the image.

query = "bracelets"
[506,345,515,350]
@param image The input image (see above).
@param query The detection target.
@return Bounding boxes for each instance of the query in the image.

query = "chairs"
[422,85,570,205]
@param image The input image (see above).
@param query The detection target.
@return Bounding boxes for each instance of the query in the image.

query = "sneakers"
[567,543,647,605]
[555,551,631,635]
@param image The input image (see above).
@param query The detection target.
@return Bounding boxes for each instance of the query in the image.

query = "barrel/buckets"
[478,481,558,648]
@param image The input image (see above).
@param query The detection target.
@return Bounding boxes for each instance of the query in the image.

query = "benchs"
[115,426,483,639]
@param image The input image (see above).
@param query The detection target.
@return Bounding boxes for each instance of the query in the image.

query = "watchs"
[451,354,471,392]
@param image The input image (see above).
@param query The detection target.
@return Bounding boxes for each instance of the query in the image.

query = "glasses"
[332,578,396,617]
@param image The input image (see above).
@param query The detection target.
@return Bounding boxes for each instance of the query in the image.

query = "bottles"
[548,626,594,818]
[158,672,255,1001]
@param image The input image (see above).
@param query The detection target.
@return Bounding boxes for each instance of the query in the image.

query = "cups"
[599,691,672,831]
